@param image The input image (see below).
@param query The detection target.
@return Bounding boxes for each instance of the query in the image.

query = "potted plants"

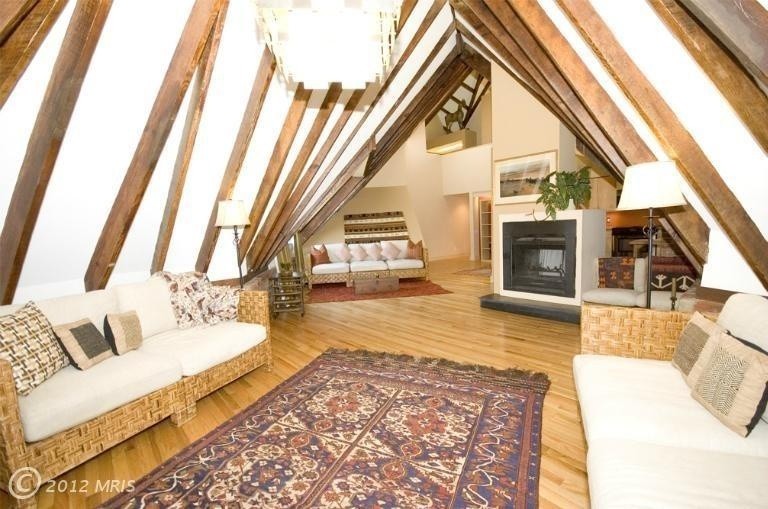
[525,165,594,222]
[277,241,294,277]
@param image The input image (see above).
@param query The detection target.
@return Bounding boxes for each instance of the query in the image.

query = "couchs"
[572,291,767,508]
[307,247,428,290]
[1,270,274,509]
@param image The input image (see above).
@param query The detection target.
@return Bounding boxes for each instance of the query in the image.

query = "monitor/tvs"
[510,233,567,291]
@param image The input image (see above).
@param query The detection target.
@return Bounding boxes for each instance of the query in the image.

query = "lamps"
[615,158,689,309]
[212,198,251,289]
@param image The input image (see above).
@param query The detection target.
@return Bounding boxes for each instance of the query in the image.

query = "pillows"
[670,309,731,390]
[151,270,213,331]
[310,238,424,267]
[688,324,767,438]
[189,285,243,327]
[51,317,115,373]
[1,299,71,399]
[103,310,144,357]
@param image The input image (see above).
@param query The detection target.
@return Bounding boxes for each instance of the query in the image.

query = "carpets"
[94,345,552,509]
[303,278,455,305]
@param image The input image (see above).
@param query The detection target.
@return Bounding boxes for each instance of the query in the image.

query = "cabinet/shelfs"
[479,199,492,263]
[268,271,306,320]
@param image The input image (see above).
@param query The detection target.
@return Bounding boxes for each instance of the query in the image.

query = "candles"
[671,277,676,298]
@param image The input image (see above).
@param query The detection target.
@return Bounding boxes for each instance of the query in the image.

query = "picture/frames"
[492,148,558,207]
